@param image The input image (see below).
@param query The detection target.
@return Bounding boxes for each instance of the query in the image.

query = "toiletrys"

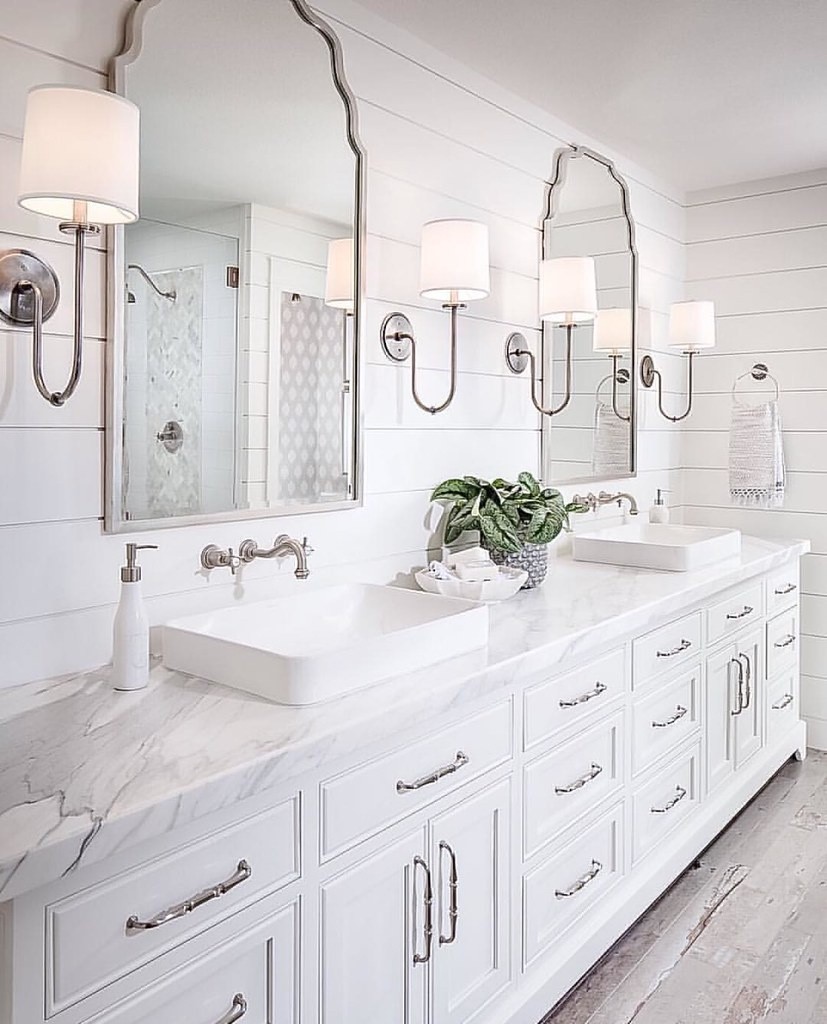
[648,488,674,524]
[111,541,160,692]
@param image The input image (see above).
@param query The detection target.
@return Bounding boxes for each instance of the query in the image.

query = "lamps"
[381,218,490,416]
[640,301,716,422]
[506,256,600,415]
[0,82,141,405]
[325,238,356,315]
[593,308,633,422]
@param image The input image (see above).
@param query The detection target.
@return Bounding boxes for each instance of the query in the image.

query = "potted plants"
[430,473,592,591]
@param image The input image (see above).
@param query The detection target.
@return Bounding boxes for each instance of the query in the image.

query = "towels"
[591,402,633,477]
[727,398,786,509]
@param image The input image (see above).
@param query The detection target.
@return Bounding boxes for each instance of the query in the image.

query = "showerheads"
[290,293,300,303]
[126,263,177,305]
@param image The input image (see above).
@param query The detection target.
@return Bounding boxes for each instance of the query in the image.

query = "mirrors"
[105,0,369,534]
[542,145,639,489]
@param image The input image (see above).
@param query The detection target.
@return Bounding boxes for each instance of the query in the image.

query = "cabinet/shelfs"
[0,553,806,1023]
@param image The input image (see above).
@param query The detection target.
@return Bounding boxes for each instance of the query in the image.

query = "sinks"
[572,521,743,571]
[163,580,491,707]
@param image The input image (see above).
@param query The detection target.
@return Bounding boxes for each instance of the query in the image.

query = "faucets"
[574,490,640,516]
[239,538,310,581]
